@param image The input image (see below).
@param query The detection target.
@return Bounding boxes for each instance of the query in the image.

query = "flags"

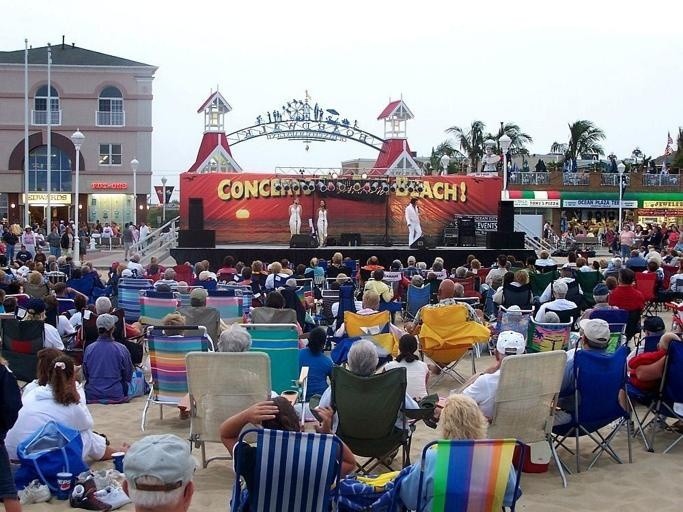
[668,132,674,144]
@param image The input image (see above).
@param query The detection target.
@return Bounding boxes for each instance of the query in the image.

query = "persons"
[460,331,524,421]
[544,214,683,257]
[219,397,356,489]
[315,201,328,247]
[4,347,130,463]
[558,319,626,416]
[612,331,680,431]
[0,250,683,404]
[481,146,501,172]
[1,363,23,512]
[218,330,278,399]
[319,340,420,465]
[405,198,423,246]
[289,197,303,237]
[123,434,199,512]
[400,395,522,512]
[0,218,170,263]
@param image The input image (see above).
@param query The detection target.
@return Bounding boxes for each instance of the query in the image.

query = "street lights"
[617,163,627,234]
[161,176,167,224]
[440,154,450,176]
[498,131,512,192]
[130,155,139,227]
[70,127,85,271]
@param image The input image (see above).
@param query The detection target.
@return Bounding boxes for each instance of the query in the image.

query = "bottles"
[244,311,251,329]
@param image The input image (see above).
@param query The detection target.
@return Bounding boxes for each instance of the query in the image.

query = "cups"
[110,451,126,472]
[56,472,72,500]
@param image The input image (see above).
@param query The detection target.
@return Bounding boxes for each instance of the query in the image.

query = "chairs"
[633,338,682,454]
[551,344,632,471]
[487,348,568,489]
[2,317,76,391]
[1,255,682,355]
[416,305,481,390]
[139,324,216,433]
[184,349,274,469]
[236,322,311,433]
[414,435,525,511]
[229,427,344,512]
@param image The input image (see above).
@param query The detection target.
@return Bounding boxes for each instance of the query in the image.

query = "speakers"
[290,234,317,248]
[342,232,360,246]
[498,200,514,232]
[189,197,203,230]
[487,231,526,249]
[175,230,216,248]
[327,238,336,246]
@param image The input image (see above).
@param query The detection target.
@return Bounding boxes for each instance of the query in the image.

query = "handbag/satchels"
[15,420,89,495]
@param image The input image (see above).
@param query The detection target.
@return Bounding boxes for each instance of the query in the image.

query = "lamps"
[275,177,424,192]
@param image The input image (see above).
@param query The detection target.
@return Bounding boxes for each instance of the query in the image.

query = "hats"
[408,256,416,264]
[123,434,196,490]
[496,282,664,356]
[25,225,32,230]
[27,269,211,333]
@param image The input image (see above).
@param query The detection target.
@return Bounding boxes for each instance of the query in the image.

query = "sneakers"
[666,419,683,431]
[18,480,50,505]
[70,470,128,510]
[646,419,665,432]
[610,417,634,434]
